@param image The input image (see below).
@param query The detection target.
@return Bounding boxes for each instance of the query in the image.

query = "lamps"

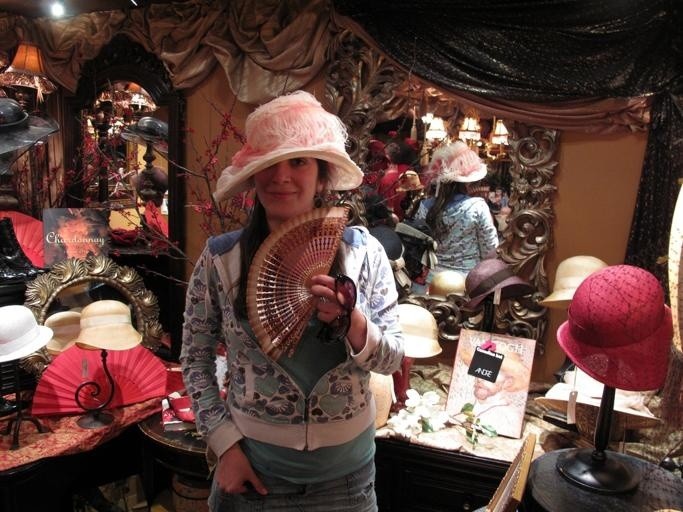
[422,102,511,162]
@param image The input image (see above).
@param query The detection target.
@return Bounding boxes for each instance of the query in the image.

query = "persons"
[407,140,499,288]
[178,89,404,512]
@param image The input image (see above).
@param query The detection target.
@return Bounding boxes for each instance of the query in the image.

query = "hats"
[44,311,81,356]
[121,116,169,155]
[537,255,610,311]
[0,304,54,363]
[367,226,413,301]
[422,270,466,307]
[361,105,436,225]
[462,258,533,313]
[75,299,143,352]
[427,141,488,184]
[396,304,443,358]
[0,97,59,155]
[393,220,435,275]
[555,264,674,392]
[210,89,365,203]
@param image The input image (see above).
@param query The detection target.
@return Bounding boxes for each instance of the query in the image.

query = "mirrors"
[323,30,560,357]
[61,35,185,267]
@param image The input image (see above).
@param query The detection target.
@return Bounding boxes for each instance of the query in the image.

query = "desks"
[0,359,512,512]
